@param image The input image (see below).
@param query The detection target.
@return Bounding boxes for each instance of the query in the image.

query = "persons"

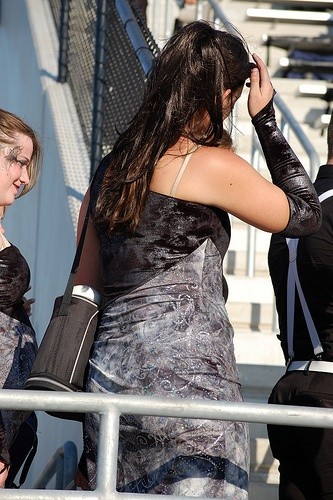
[73,19,323,500]
[0,106,44,490]
[267,109,333,500]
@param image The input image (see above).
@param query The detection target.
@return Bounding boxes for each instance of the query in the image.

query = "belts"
[286,360,333,373]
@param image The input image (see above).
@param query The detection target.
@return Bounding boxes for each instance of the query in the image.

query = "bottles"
[72,285,100,306]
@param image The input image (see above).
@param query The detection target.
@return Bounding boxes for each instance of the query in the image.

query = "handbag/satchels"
[26,295,102,423]
